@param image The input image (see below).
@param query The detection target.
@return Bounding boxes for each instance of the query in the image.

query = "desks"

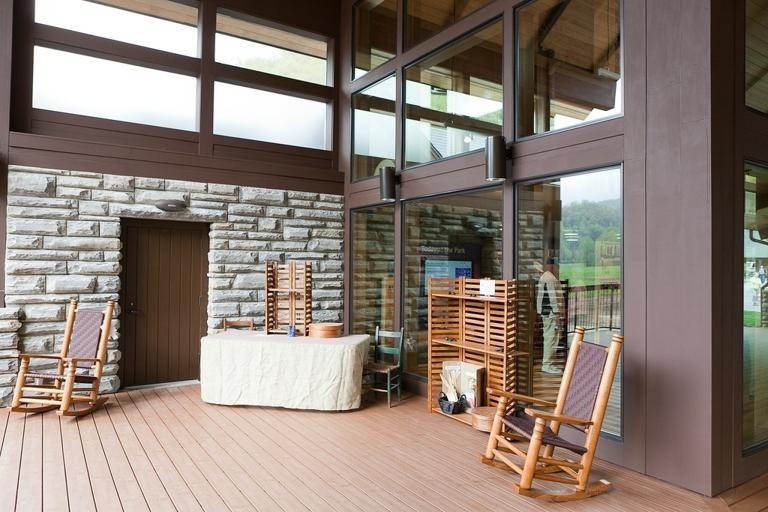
[201,331,370,411]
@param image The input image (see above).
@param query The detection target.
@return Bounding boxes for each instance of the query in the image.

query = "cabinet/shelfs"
[425,275,518,435]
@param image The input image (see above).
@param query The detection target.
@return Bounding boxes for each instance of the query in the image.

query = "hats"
[546,257,560,265]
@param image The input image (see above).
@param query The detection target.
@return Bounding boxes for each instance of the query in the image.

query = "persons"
[537,258,565,375]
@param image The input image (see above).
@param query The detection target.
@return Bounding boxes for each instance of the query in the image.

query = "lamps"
[379,166,396,202]
[485,135,507,183]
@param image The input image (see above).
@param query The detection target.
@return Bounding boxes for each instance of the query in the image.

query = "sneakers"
[541,365,561,374]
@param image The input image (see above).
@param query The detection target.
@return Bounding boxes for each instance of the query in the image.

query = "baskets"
[438,391,466,415]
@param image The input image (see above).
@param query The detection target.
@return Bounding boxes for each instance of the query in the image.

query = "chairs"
[223,317,255,331]
[479,325,625,502]
[363,326,406,409]
[5,299,117,417]
